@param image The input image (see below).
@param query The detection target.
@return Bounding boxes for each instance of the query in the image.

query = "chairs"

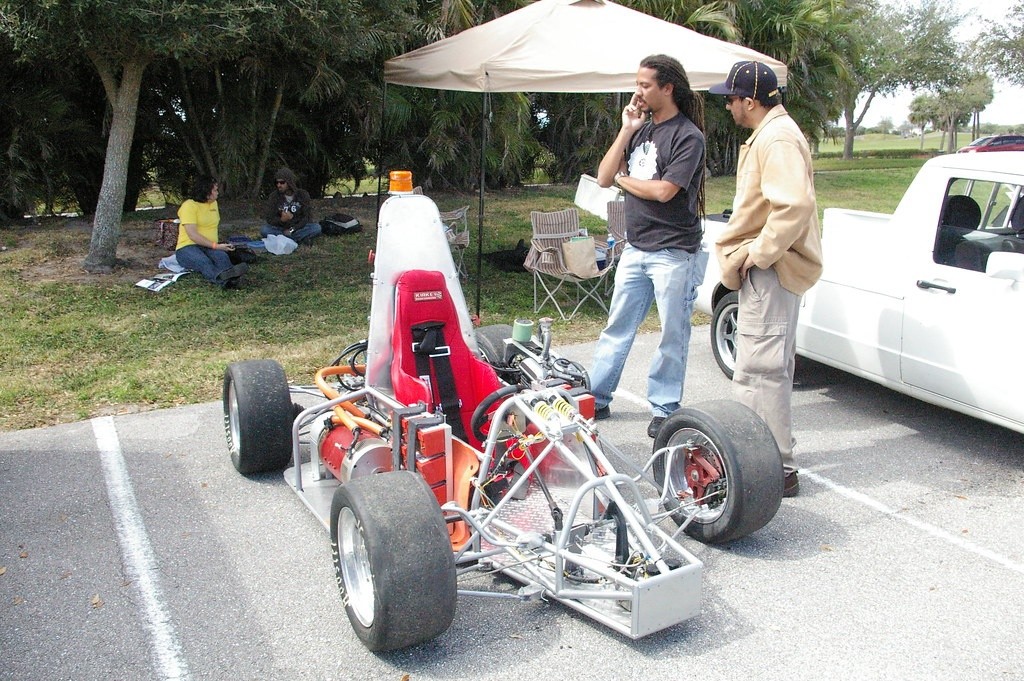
[391,271,542,476]
[1002,195,1024,254]
[413,185,470,278]
[935,194,993,273]
[524,209,608,320]
[595,201,627,296]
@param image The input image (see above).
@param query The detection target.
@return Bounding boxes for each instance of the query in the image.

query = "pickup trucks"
[691,133,1023,434]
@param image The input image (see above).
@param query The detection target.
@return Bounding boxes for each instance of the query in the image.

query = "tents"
[377,0,788,326]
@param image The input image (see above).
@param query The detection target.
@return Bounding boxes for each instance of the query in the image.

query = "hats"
[709,60,778,99]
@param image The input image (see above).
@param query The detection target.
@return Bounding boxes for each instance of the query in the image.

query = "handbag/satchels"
[228,246,256,264]
[563,233,599,276]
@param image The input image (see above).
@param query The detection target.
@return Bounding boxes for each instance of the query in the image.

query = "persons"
[708,61,824,497]
[587,54,708,438]
[260,167,322,243]
[175,175,248,289]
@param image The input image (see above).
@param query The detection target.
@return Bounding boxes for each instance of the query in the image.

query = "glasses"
[726,96,741,104]
[275,181,286,184]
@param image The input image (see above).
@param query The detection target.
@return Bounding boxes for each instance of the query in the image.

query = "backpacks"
[319,213,362,235]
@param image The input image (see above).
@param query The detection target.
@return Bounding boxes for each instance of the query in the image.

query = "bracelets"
[213,242,216,249]
[615,172,628,182]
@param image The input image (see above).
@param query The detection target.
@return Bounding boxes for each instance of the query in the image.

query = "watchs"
[289,227,294,233]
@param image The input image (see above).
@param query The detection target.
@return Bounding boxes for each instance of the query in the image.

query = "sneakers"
[648,416,665,438]
[595,406,611,419]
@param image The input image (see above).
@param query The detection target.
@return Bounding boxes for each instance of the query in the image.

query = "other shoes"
[783,471,799,497]
[219,263,248,280]
[223,277,246,288]
